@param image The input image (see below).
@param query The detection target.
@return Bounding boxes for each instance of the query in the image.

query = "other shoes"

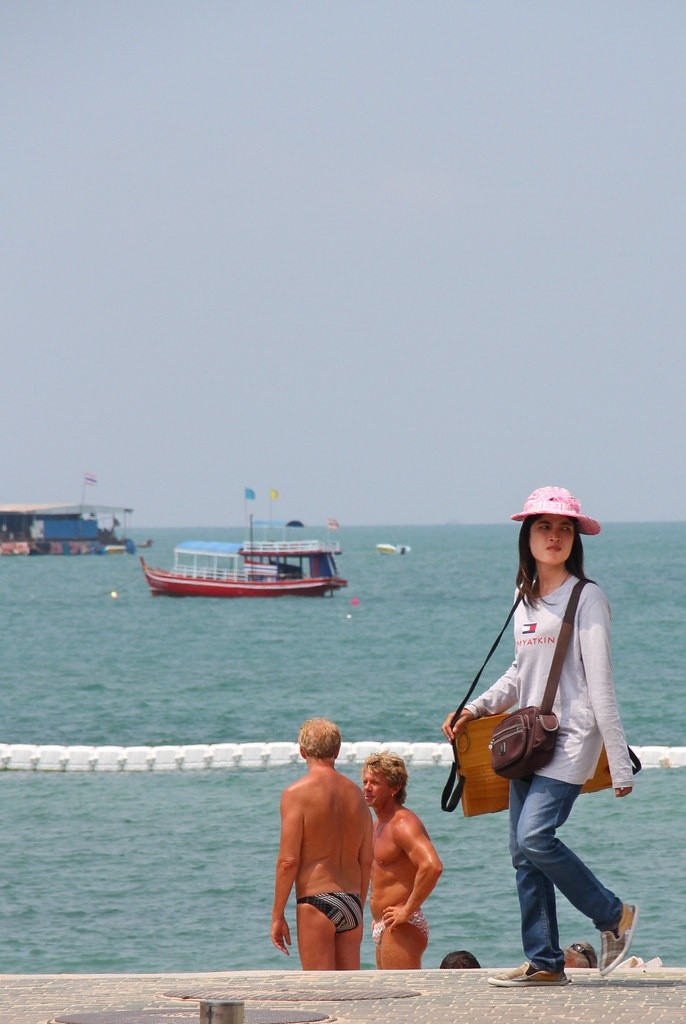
[599,903,637,976]
[488,961,569,985]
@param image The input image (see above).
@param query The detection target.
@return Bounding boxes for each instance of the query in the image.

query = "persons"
[440,486,639,987]
[360,752,442,970]
[441,950,481,968]
[270,717,373,971]
[562,942,598,969]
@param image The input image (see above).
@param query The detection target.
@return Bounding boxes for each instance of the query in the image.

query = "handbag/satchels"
[489,706,559,779]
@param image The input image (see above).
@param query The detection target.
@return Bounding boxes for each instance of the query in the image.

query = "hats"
[511,487,600,535]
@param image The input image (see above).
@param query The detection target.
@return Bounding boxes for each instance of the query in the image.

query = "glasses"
[571,943,592,969]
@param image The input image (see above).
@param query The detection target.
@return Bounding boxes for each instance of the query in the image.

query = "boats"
[141,512,348,597]
[377,544,412,556]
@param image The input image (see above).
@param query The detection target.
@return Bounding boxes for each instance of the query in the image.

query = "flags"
[270,489,278,499]
[328,519,339,530]
[84,473,97,487]
[244,487,256,499]
